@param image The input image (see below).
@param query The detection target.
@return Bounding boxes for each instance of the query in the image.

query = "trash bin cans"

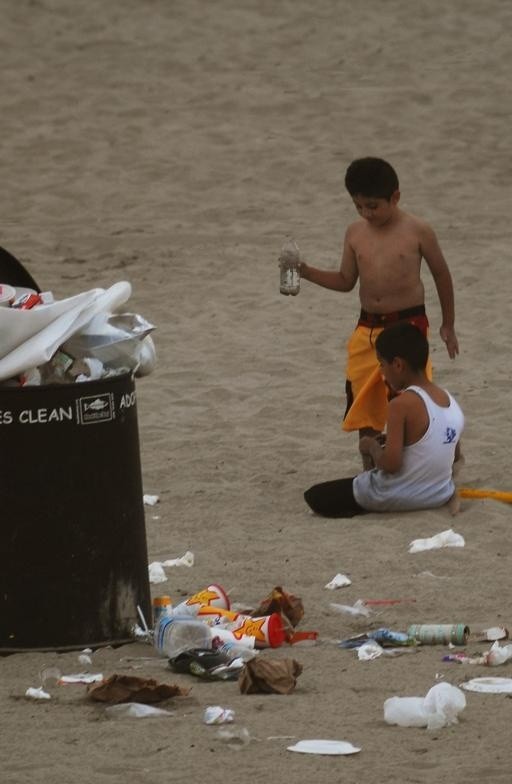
[0,371,152,652]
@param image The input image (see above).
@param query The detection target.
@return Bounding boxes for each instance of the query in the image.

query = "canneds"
[169,646,244,682]
[406,623,469,646]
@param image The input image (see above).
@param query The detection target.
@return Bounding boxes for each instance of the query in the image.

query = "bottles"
[153,617,221,658]
[279,233,302,298]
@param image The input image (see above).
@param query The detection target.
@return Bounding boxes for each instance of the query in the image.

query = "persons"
[304,324,465,518]
[278,158,459,472]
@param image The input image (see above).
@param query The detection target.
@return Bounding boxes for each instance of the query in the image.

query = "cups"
[168,584,285,649]
[1,282,18,306]
